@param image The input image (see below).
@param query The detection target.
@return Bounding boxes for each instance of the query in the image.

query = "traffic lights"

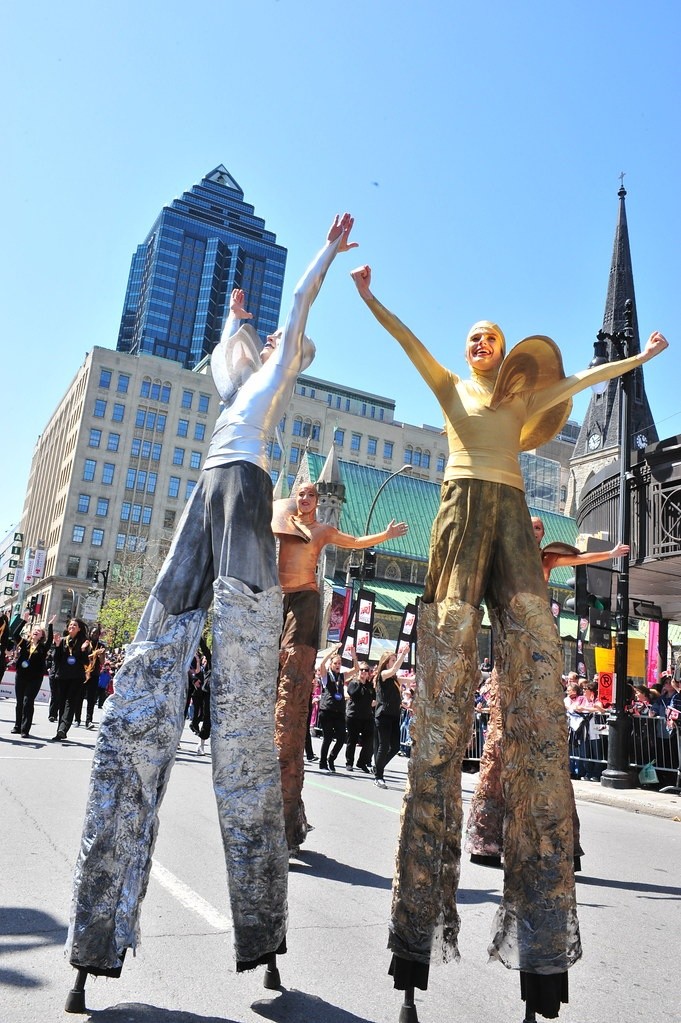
[591,595,610,613]
[364,550,377,580]
[350,566,360,578]
[567,565,589,616]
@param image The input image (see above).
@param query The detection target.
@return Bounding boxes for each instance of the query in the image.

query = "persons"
[271,484,409,856]
[463,657,493,774]
[561,671,612,782]
[624,657,681,790]
[0,610,125,740]
[303,642,416,789]
[64,212,359,1013]
[351,263,670,1023]
[471,518,629,873]
[176,637,211,756]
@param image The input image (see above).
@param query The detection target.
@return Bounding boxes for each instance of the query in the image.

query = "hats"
[664,677,671,682]
[298,482,318,500]
[467,320,506,359]
[301,335,316,373]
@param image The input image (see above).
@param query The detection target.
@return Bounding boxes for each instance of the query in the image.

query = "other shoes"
[356,764,376,773]
[48,717,57,722]
[374,779,387,789]
[581,776,590,781]
[73,721,79,728]
[346,764,353,771]
[21,733,29,738]
[11,729,20,734]
[328,758,335,772]
[319,766,330,771]
[52,736,67,741]
[98,707,102,709]
[86,722,94,730]
[590,777,599,782]
[308,757,319,762]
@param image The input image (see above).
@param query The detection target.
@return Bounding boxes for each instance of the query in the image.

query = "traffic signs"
[634,601,663,619]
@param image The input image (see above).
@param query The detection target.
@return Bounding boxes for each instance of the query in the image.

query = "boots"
[198,737,205,756]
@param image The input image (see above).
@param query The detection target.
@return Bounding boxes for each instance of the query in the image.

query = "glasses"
[583,688,591,690]
[360,669,369,672]
[664,682,671,685]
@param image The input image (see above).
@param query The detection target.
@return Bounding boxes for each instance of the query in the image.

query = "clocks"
[637,434,648,449]
[589,433,602,448]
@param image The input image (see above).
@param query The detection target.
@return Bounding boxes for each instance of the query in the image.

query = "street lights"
[360,464,413,589]
[26,601,37,634]
[92,569,108,629]
[590,330,635,788]
